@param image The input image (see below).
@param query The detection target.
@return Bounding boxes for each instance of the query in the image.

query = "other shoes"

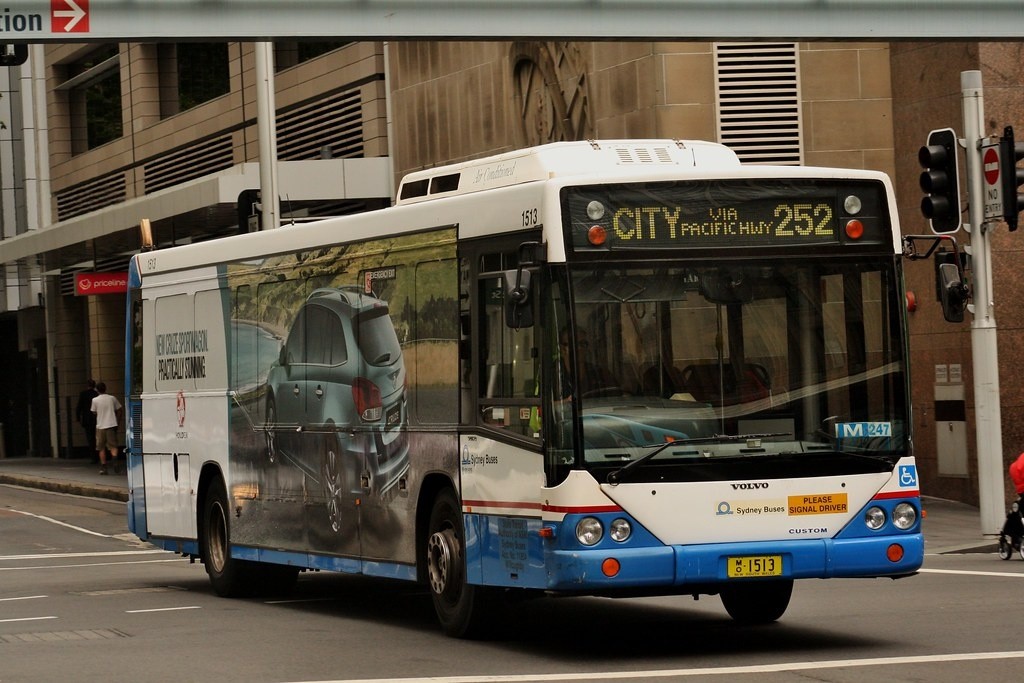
[115,464,121,474]
[99,468,107,475]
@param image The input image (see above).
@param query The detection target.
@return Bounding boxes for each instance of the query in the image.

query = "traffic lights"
[1001,125,1024,232]
[918,127,962,234]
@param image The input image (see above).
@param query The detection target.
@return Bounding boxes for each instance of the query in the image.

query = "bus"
[124,139,926,637]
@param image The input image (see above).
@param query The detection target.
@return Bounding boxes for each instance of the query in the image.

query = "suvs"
[266,285,409,538]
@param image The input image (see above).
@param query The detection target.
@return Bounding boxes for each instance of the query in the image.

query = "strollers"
[998,496,1024,560]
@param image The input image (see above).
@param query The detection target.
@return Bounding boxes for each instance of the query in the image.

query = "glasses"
[561,340,588,353]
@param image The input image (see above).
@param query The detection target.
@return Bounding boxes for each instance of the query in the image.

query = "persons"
[75,379,99,465]
[548,324,623,420]
[1008,451,1024,501]
[132,376,143,399]
[90,383,122,475]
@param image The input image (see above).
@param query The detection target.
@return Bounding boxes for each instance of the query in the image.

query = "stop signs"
[984,148,999,185]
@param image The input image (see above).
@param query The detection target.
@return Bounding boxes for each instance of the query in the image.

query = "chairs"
[634,361,771,411]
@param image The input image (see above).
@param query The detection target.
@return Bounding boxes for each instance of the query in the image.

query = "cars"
[229,318,289,454]
